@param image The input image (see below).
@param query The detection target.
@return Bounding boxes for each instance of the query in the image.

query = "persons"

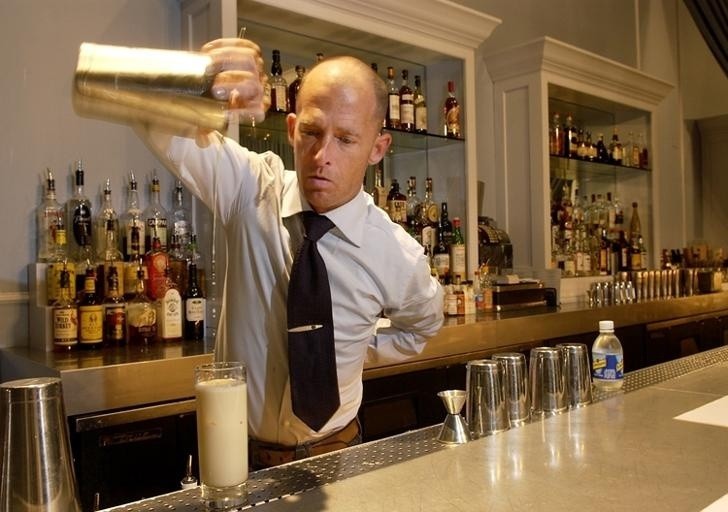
[129,38,443,474]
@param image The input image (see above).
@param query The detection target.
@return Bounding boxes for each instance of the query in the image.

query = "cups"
[194,360,248,498]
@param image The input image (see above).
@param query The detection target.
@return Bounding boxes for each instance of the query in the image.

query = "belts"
[246,419,359,465]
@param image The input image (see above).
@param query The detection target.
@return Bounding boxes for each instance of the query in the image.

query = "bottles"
[183,263,205,339]
[370,63,387,128]
[79,269,105,349]
[639,133,649,170]
[128,272,157,345]
[433,219,450,285]
[582,227,591,276]
[444,286,456,317]
[600,230,607,271]
[289,66,310,115]
[94,179,119,261]
[483,267,494,313]
[562,187,572,226]
[453,276,464,316]
[186,236,205,298]
[614,197,623,225]
[386,67,401,130]
[104,267,131,347]
[316,54,323,65]
[474,273,484,312]
[573,191,582,228]
[399,70,415,133]
[101,224,125,302]
[630,234,640,270]
[550,113,564,156]
[606,192,617,231]
[587,131,596,160]
[596,133,607,162]
[444,81,461,139]
[422,178,438,228]
[439,203,453,246]
[609,128,622,164]
[168,237,188,298]
[590,194,600,233]
[387,180,407,226]
[157,268,183,343]
[574,230,583,276]
[372,172,386,211]
[563,237,575,278]
[52,272,78,353]
[406,176,421,227]
[626,131,634,166]
[577,130,587,160]
[551,230,564,278]
[565,113,577,159]
[144,176,169,259]
[451,219,466,284]
[464,279,476,316]
[122,173,146,263]
[415,203,434,257]
[38,168,65,264]
[268,50,288,113]
[67,159,94,261]
[638,236,647,270]
[588,232,600,275]
[168,180,194,255]
[126,228,144,299]
[632,139,640,167]
[414,76,427,134]
[76,238,97,302]
[146,238,169,299]
[597,194,608,231]
[630,203,641,247]
[619,232,629,271]
[592,321,623,391]
[47,226,77,306]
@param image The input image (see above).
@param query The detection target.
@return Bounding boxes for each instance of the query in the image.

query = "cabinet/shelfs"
[179,0,501,337]
[483,35,677,308]
[1,290,727,435]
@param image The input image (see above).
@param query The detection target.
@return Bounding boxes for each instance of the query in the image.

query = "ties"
[286,212,342,436]
